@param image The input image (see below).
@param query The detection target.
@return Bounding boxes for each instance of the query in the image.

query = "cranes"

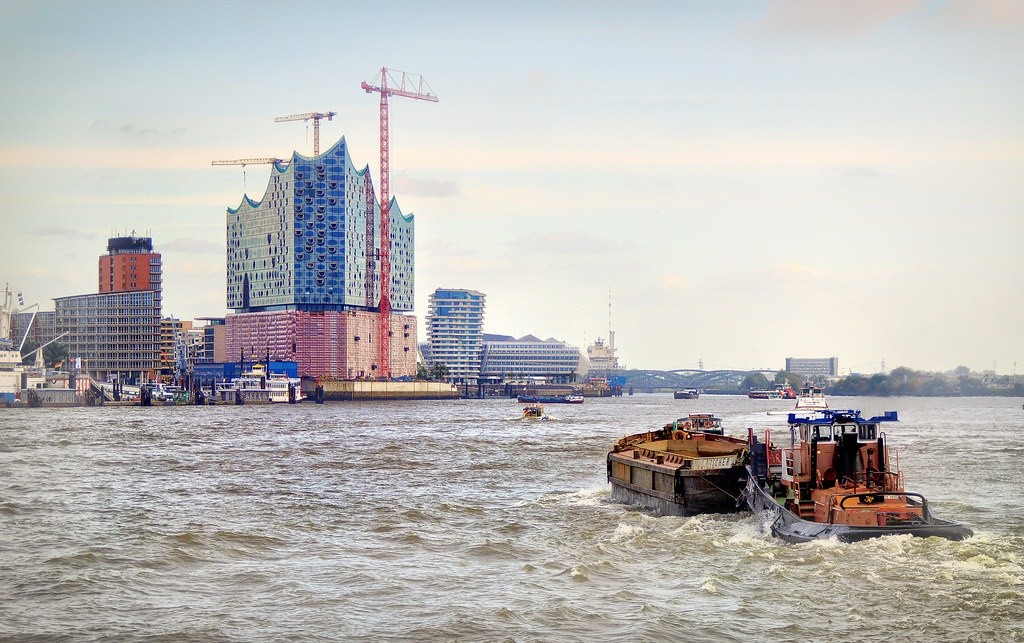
[0,282,22,309]
[273,110,339,161]
[360,65,440,380]
[211,157,280,198]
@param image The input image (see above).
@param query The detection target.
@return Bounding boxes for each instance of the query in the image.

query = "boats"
[517,393,584,404]
[676,413,724,437]
[605,422,789,518]
[674,387,700,400]
[793,382,829,410]
[748,384,797,399]
[742,410,973,546]
[522,403,548,422]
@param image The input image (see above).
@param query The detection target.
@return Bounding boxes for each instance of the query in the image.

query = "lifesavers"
[705,421,712,428]
[672,430,687,441]
[684,421,691,429]
